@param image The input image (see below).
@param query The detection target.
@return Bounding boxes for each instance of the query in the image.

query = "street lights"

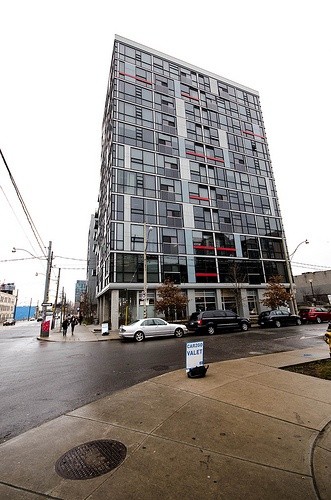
[308,278,317,307]
[281,234,309,316]
[12,241,52,321]
[35,267,61,329]
[142,222,153,318]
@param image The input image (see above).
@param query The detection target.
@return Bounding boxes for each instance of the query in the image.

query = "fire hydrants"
[323,322,331,358]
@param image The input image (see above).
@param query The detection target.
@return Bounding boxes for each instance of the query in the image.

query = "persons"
[62,314,83,336]
[250,309,256,315]
[308,301,315,307]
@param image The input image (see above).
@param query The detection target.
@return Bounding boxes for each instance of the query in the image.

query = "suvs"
[298,306,331,324]
[257,309,302,328]
[188,310,251,336]
[37,316,43,322]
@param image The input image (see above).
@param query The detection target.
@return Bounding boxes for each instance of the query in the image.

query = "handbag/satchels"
[76,321,78,325]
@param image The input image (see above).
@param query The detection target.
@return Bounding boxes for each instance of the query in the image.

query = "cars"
[2,318,16,326]
[30,318,35,321]
[119,318,189,342]
